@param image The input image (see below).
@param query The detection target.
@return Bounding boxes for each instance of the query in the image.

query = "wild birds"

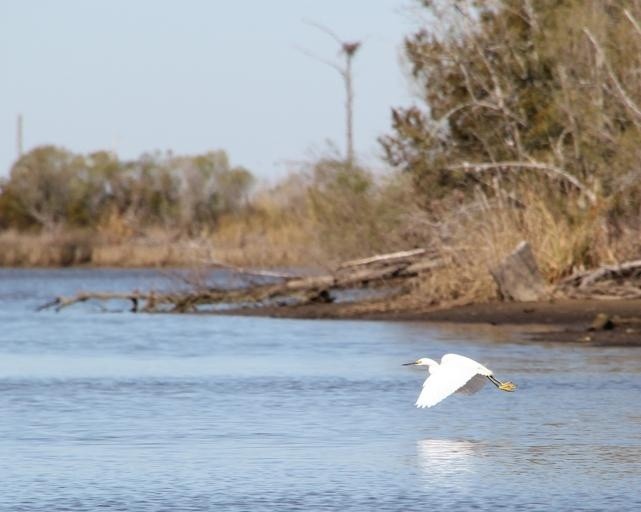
[402,353,518,408]
